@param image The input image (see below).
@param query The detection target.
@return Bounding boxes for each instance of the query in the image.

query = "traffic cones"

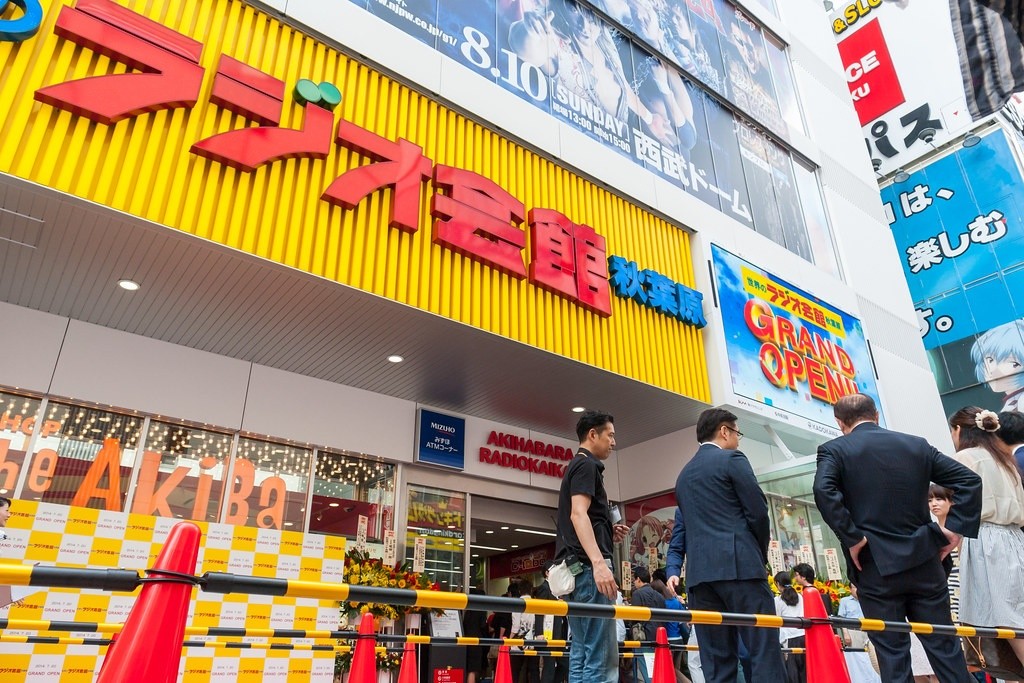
[347,611,378,683]
[648,627,678,683]
[96,522,201,683]
[802,587,853,683]
[493,636,514,683]
[397,636,420,683]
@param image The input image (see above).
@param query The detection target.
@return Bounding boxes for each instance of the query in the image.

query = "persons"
[666,409,789,683]
[613,567,753,683]
[553,412,631,683]
[464,562,573,683]
[812,394,983,683]
[929,405,1024,683]
[773,563,882,683]
[506,0,782,161]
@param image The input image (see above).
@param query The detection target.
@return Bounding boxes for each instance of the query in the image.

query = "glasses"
[719,424,744,440]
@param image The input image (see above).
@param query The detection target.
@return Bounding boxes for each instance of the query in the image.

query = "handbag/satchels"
[547,559,575,597]
[960,622,1024,681]
[632,623,646,641]
